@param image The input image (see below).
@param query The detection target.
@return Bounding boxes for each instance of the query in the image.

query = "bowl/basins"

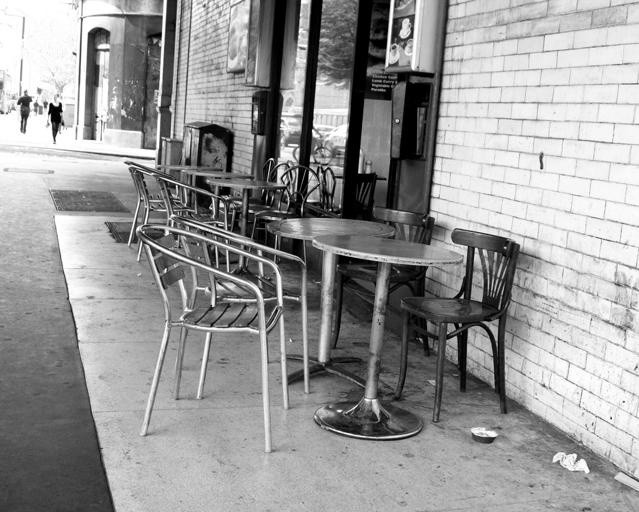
[470,426,498,444]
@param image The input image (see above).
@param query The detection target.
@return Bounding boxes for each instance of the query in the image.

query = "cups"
[388,18,415,65]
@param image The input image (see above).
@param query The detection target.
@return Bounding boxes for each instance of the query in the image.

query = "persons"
[311,126,323,154]
[17,87,65,144]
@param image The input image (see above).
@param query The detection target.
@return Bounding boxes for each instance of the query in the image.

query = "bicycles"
[293,134,333,166]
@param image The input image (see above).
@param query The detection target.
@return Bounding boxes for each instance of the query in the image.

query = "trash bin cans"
[161,137,183,187]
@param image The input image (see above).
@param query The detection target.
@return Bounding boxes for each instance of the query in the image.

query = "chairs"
[125,158,521,455]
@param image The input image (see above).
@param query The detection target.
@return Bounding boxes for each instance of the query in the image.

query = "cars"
[280,111,350,159]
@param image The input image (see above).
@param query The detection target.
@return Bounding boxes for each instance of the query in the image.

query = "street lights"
[4,11,26,103]
